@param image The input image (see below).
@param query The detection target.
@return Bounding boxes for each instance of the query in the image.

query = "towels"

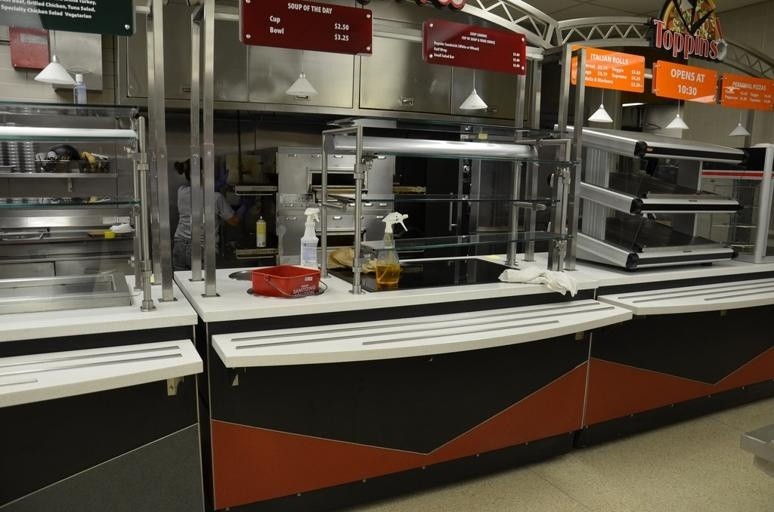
[495,255,579,302]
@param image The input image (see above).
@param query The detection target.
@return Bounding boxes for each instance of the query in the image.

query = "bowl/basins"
[35,144,113,172]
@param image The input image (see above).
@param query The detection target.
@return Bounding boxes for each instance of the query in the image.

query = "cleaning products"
[299,206,320,271]
[374,213,409,287]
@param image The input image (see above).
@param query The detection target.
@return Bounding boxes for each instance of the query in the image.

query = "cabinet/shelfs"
[453,35,533,122]
[318,126,577,295]
[359,29,451,114]
[0,107,156,313]
[248,20,357,108]
[126,11,246,102]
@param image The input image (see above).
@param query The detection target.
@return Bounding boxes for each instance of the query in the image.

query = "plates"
[0,141,36,173]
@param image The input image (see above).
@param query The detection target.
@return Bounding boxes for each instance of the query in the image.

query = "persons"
[172,157,241,270]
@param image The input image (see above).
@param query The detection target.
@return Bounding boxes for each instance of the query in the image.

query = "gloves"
[235,196,254,218]
[214,167,229,187]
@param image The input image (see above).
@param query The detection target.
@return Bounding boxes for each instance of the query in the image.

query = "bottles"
[74,74,88,104]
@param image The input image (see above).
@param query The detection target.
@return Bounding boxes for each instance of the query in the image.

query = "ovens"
[231,147,395,258]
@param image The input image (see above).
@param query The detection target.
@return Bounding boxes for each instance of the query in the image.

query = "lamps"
[283,49,489,114]
[31,1,74,86]
[587,77,751,140]
